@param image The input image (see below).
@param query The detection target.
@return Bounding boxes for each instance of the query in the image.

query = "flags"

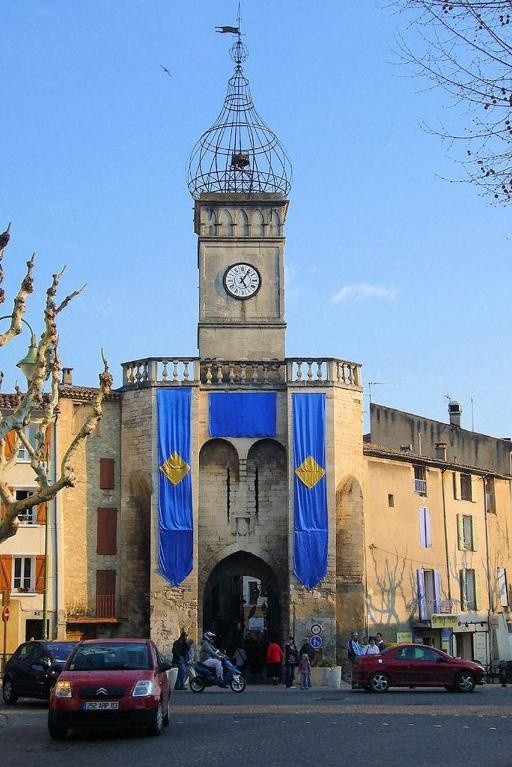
[214,24,241,35]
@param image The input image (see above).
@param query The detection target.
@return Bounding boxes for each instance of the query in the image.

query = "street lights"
[0,316,41,383]
[479,472,499,611]
[440,466,453,612]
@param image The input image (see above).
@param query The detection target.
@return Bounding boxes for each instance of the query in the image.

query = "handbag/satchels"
[243,660,250,667]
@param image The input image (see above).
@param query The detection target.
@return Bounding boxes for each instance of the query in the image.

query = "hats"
[181,632,188,636]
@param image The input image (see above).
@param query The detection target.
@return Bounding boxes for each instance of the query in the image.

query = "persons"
[347,631,384,657]
[298,636,316,687]
[171,631,192,691]
[296,653,312,690]
[283,635,300,689]
[183,639,196,689]
[216,633,284,685]
[198,631,228,688]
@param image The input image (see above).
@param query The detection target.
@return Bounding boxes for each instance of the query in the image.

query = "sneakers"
[175,687,187,690]
[272,678,312,690]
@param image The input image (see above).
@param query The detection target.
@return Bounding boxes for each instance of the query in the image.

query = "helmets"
[204,632,216,644]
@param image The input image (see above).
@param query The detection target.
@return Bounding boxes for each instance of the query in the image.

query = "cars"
[3,640,80,704]
[351,643,484,694]
[48,638,172,736]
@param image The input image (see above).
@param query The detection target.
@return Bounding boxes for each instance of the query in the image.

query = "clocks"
[223,263,262,301]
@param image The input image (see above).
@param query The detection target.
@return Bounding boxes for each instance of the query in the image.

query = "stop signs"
[2,608,9,623]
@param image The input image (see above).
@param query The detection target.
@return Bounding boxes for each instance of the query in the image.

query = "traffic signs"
[310,624,324,636]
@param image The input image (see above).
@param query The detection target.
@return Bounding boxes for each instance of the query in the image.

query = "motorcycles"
[188,649,247,693]
